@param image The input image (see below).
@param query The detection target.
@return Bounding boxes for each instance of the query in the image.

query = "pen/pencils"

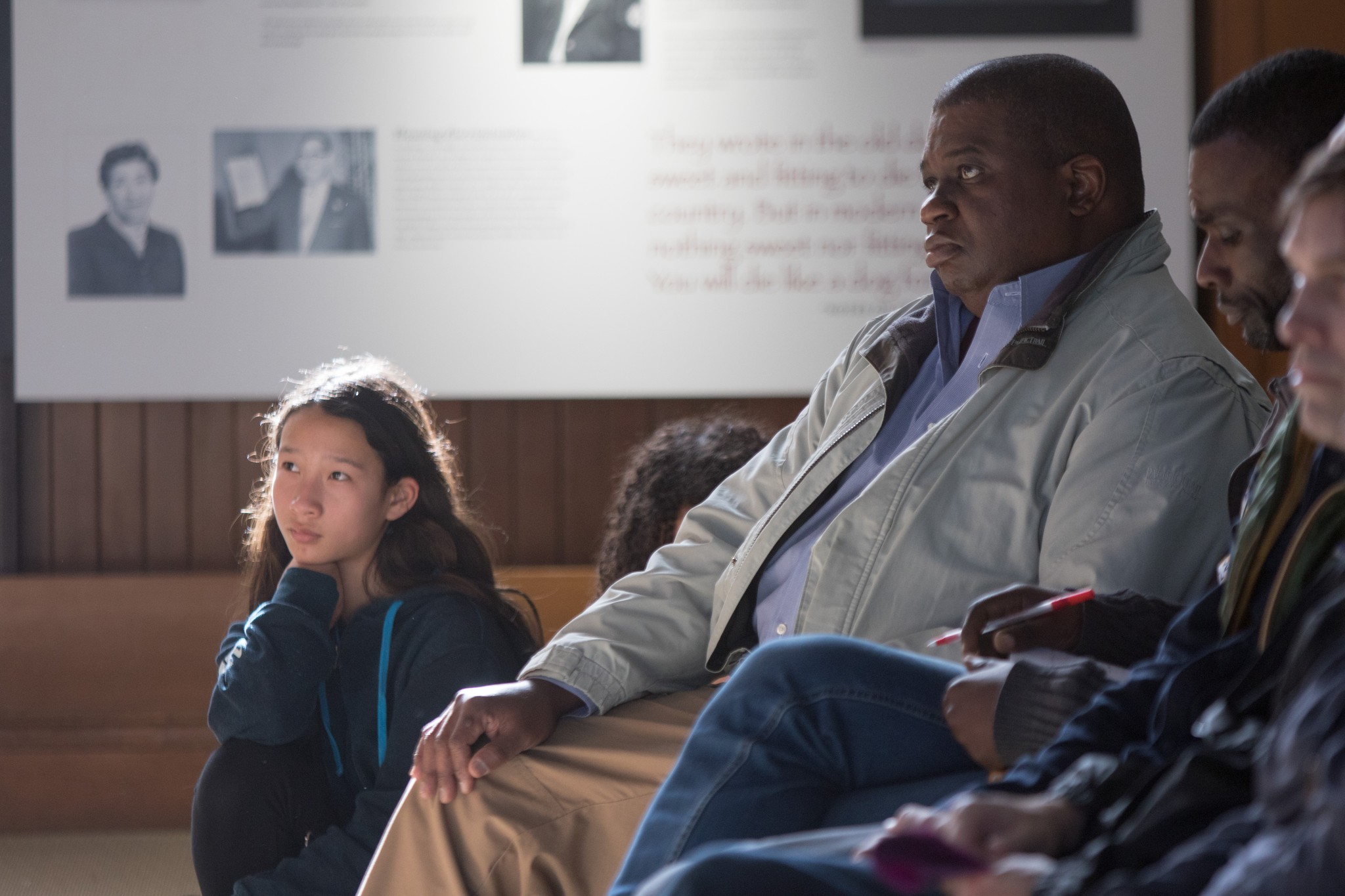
[926,588,1095,649]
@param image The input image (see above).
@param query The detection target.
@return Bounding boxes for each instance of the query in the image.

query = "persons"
[593,412,769,597]
[608,45,1345,896]
[191,355,544,896]
[63,138,184,297]
[218,131,368,253]
[357,50,1273,896]
